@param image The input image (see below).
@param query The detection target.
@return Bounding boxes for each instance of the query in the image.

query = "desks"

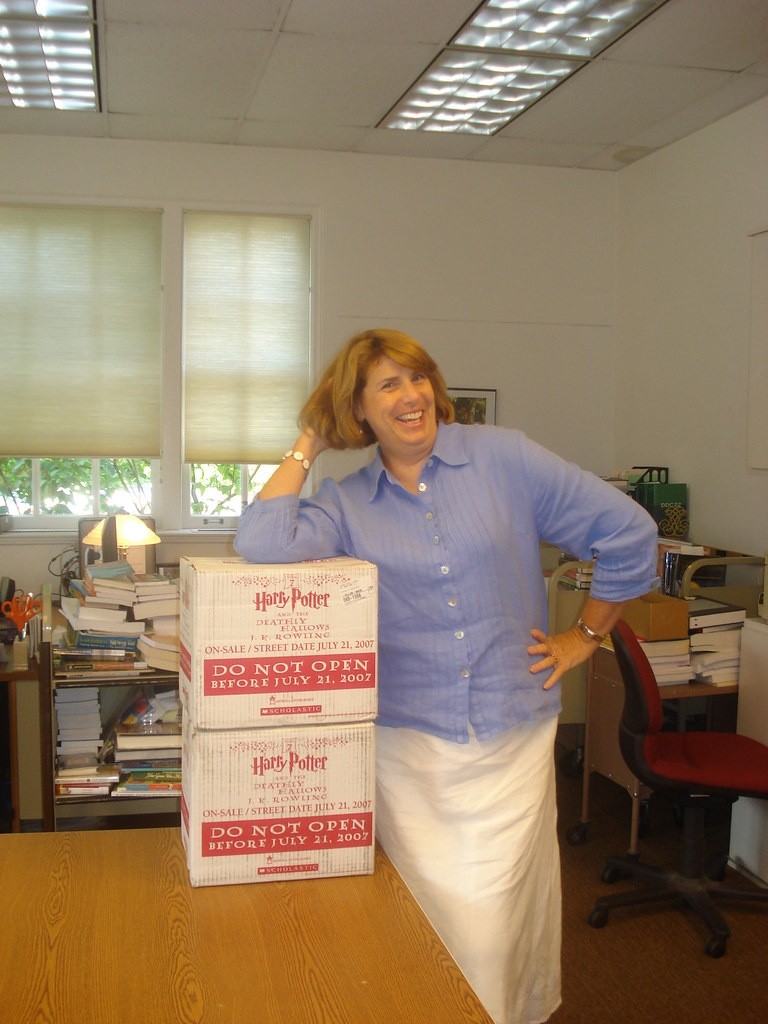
[0,655,38,833]
[581,647,738,855]
[0,828,493,1024]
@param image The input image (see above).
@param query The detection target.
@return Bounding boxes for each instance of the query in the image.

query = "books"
[633,596,750,689]
[55,555,182,799]
[29,606,43,661]
[549,534,710,593]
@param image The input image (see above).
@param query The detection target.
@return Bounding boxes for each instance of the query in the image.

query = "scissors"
[2,595,43,643]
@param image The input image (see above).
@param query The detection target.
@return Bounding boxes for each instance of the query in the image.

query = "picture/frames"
[446,389,497,425]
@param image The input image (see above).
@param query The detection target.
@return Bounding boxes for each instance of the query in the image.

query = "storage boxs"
[179,706,376,888]
[179,554,379,730]
[624,593,690,640]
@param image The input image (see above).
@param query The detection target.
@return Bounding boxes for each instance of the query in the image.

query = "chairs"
[588,619,768,959]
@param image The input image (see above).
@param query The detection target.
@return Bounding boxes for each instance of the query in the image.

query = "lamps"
[81,515,161,561]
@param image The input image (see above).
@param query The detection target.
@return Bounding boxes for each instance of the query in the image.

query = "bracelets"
[279,449,313,474]
[574,616,607,644]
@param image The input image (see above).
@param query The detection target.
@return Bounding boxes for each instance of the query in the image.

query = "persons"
[232,331,663,1023]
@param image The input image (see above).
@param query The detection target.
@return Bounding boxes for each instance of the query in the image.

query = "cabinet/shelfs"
[39,581,181,833]
